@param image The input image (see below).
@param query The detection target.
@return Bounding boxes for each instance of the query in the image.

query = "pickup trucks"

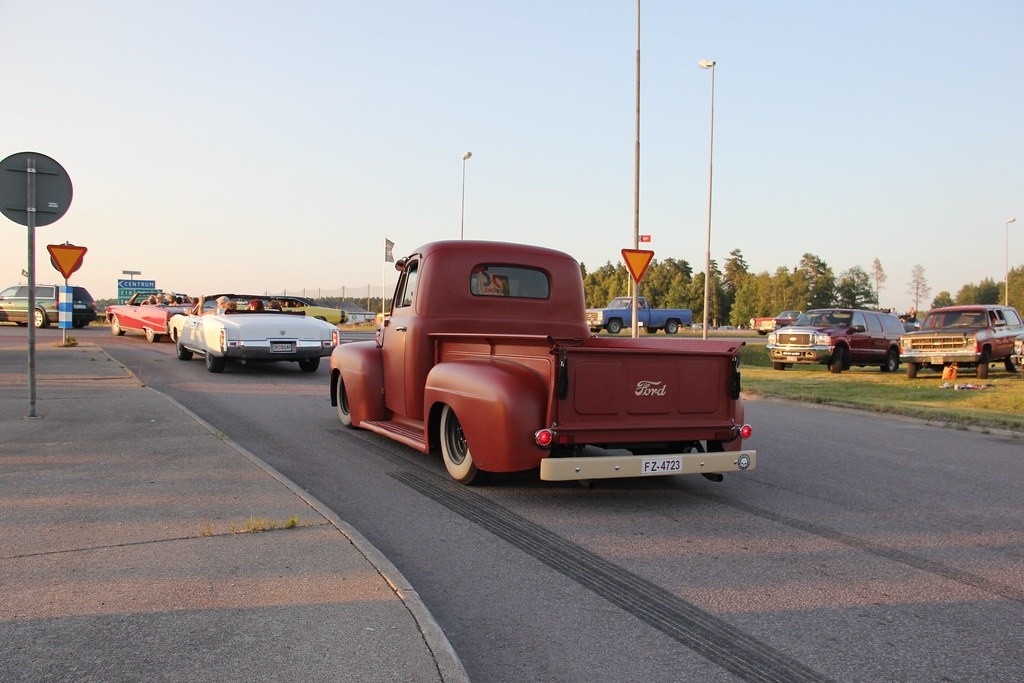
[581,294,694,336]
[328,240,757,484]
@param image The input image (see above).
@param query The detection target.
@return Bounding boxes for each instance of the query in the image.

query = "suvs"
[767,307,907,374]
[0,285,99,330]
[898,303,1023,380]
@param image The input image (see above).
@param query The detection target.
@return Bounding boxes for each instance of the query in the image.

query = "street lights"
[459,151,475,240]
[1005,216,1016,308]
[698,59,717,342]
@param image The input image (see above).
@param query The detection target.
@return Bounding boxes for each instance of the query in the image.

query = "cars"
[889,313,928,334]
[691,321,733,332]
[750,309,805,335]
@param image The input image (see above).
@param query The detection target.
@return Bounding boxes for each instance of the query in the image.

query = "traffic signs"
[117,279,156,290]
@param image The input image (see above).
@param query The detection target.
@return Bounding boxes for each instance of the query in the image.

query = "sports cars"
[236,294,350,326]
[105,292,194,343]
[171,293,341,373]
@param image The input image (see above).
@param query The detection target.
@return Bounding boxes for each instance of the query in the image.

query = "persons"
[638,301,642,308]
[249,299,264,311]
[149,292,191,306]
[899,310,916,323]
[269,300,283,311]
[192,295,237,316]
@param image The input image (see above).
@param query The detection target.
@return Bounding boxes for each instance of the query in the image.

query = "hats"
[216,296,229,303]
[156,293,166,299]
